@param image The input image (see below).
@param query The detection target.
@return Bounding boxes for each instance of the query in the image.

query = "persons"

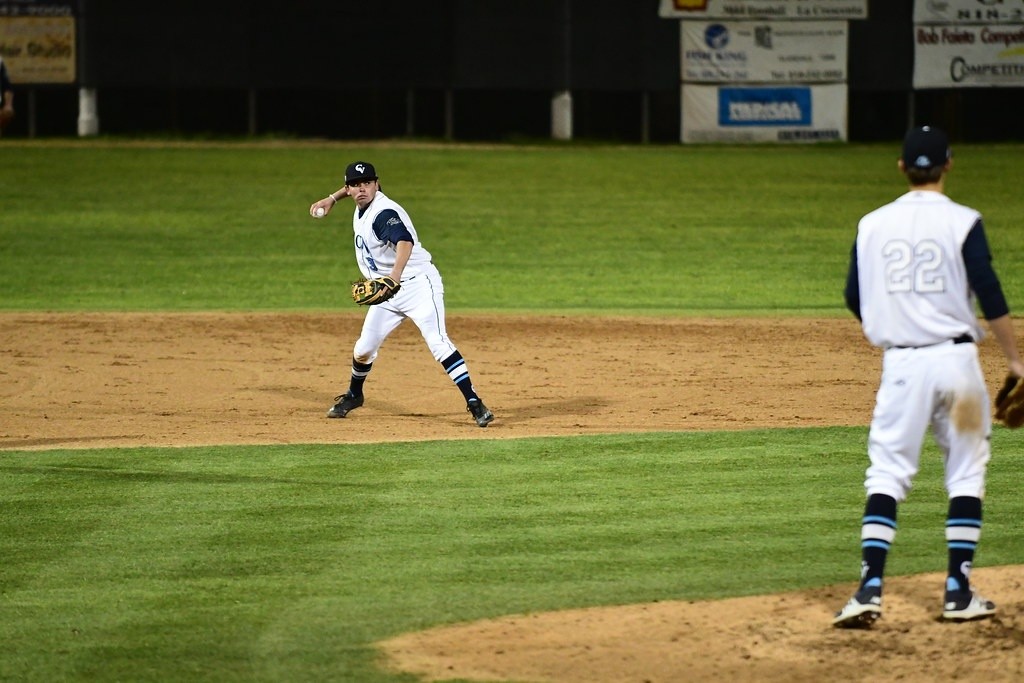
[307,161,495,429]
[0,57,15,117]
[829,126,1024,630]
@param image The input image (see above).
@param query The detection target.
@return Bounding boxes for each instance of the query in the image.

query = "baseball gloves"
[991,373,1024,429]
[349,275,402,306]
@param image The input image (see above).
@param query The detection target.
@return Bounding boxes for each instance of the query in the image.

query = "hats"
[902,123,949,169]
[344,161,379,185]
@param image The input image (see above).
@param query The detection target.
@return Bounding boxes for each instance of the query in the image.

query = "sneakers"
[942,585,998,622]
[467,398,495,427]
[832,586,882,630]
[326,389,364,419]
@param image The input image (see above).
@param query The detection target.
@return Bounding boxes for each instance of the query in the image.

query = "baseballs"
[311,207,325,220]
